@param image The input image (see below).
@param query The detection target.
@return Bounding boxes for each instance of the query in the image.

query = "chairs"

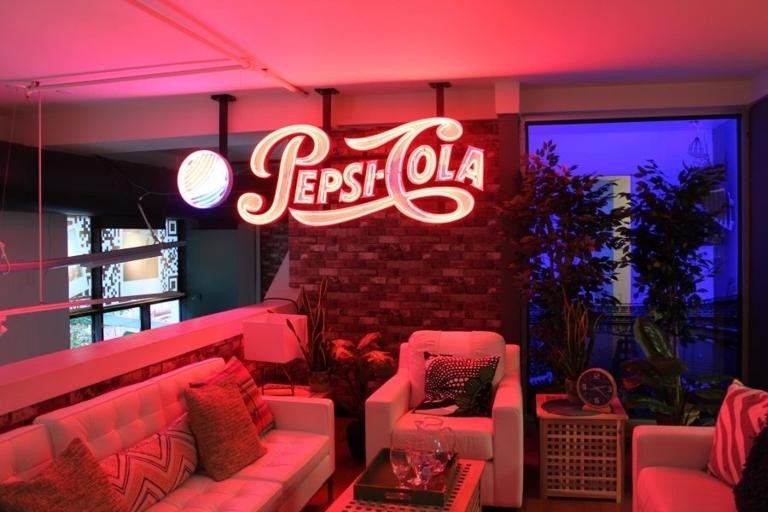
[364,343,524,508]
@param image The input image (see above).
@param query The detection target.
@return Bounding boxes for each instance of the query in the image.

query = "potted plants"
[330,332,397,463]
[547,290,608,403]
[617,315,734,425]
[285,277,333,393]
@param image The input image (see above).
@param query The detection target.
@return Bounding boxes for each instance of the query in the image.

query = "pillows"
[203,355,274,436]
[706,379,768,488]
[410,330,506,409]
[0,438,134,512]
[100,412,198,512]
[413,351,500,415]
[184,376,267,483]
[732,427,768,512]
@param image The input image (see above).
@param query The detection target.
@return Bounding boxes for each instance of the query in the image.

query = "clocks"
[577,369,617,413]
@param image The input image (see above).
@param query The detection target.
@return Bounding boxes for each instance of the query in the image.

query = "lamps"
[242,314,309,385]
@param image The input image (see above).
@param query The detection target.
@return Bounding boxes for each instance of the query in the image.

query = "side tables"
[259,384,332,397]
[534,393,628,505]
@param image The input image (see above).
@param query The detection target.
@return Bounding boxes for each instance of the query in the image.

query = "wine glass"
[388,447,435,488]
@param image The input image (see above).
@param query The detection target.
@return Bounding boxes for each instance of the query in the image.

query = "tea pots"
[414,415,457,476]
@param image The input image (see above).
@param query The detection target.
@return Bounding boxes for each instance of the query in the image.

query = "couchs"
[632,426,739,512]
[0,355,336,512]
